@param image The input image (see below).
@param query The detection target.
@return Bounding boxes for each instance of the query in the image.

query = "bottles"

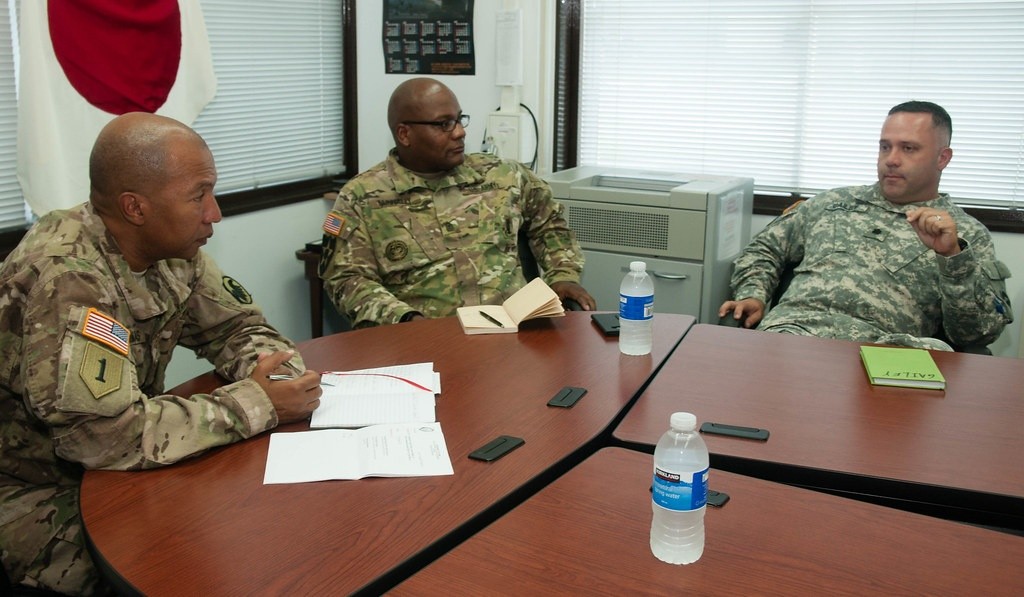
[619,260,655,355]
[649,412,709,565]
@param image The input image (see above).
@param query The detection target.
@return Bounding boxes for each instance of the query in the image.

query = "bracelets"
[400,312,416,322]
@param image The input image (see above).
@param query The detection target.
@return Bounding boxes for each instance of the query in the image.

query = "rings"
[936,216,941,221]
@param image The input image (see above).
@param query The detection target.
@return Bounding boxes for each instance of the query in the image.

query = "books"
[859,346,946,390]
[456,277,566,335]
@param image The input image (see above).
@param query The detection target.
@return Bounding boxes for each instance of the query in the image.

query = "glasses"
[402,114,470,132]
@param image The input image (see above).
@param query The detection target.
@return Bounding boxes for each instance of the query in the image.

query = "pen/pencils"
[478,310,504,329]
[266,375,337,388]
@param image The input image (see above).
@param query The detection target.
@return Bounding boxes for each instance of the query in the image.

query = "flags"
[17,0,217,219]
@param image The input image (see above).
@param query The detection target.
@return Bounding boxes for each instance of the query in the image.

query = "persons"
[718,101,1014,352]
[0,112,323,596]
[318,78,596,326]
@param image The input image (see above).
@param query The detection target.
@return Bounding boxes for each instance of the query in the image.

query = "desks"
[71,309,1024,597]
